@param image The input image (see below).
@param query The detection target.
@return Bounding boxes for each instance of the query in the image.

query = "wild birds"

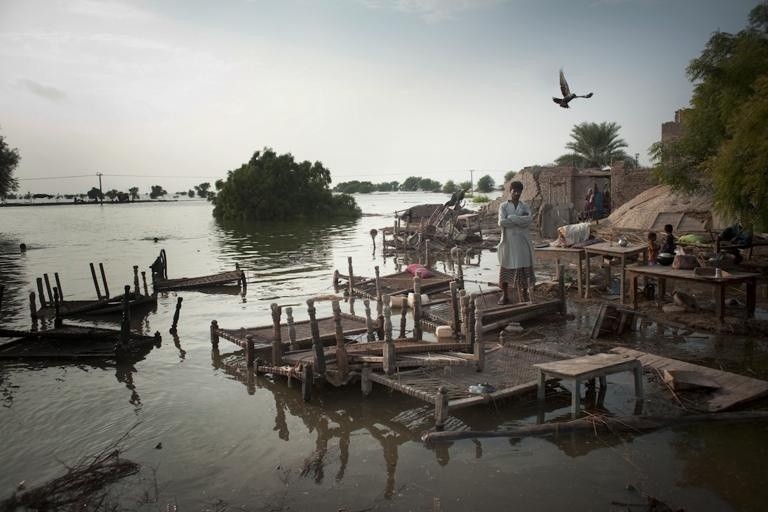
[552,70,593,109]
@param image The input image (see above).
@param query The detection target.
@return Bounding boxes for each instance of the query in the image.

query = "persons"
[583,188,610,221]
[496,181,537,305]
[648,232,660,266]
[660,224,674,255]
